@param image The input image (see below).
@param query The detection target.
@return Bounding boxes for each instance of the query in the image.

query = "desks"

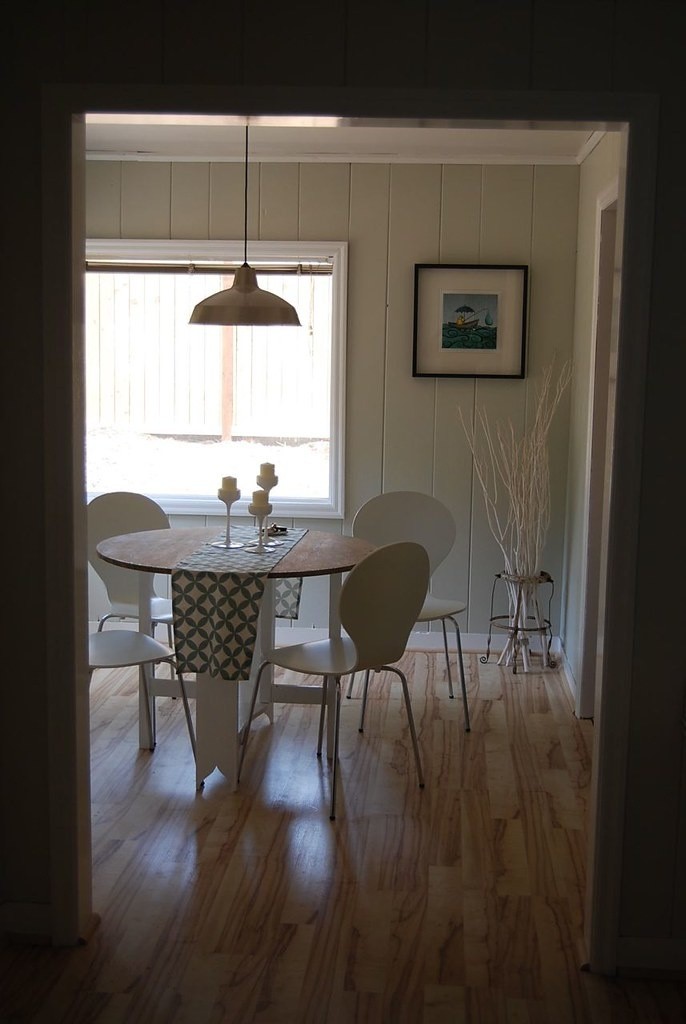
[96,525,380,751]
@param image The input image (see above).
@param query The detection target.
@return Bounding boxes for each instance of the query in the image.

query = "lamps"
[188,126,303,327]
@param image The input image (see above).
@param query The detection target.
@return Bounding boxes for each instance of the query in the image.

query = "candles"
[252,490,269,507]
[260,462,275,478]
[222,476,237,492]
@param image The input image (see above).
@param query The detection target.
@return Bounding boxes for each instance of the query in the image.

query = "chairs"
[86,490,472,819]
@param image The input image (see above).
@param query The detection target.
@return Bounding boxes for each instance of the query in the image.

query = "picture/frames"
[412,263,529,379]
